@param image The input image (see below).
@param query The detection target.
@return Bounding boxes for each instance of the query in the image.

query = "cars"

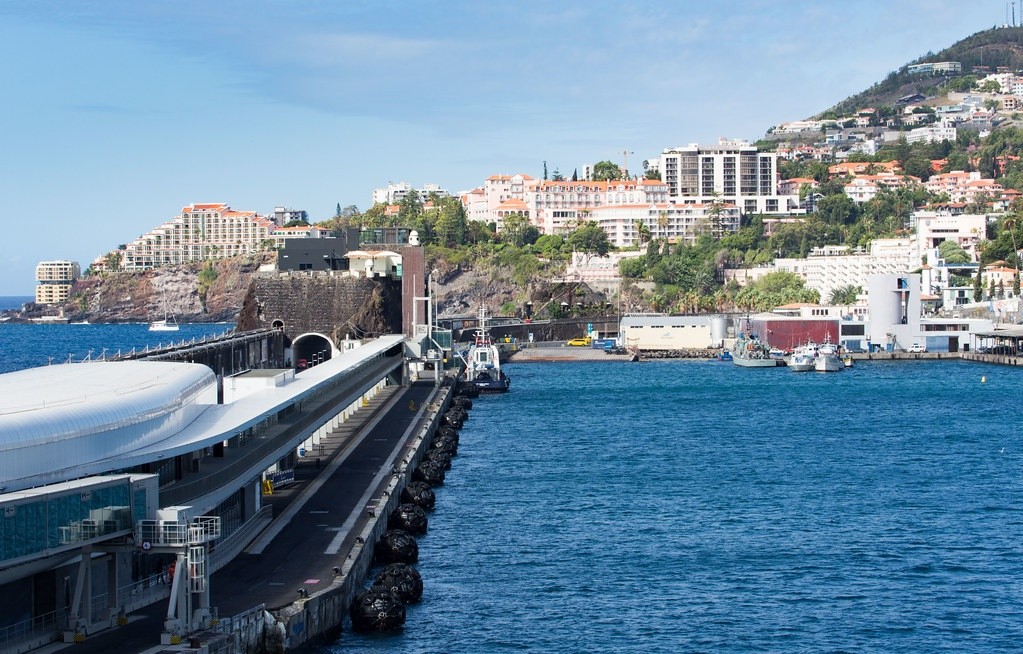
[567,335,591,347]
[976,345,1011,354]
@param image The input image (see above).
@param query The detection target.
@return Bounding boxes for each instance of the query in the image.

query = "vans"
[907,344,926,353]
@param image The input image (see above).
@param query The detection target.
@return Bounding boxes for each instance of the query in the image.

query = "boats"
[717,312,854,373]
[459,301,512,394]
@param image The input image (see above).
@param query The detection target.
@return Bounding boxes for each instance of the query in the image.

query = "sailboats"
[148,289,180,332]
